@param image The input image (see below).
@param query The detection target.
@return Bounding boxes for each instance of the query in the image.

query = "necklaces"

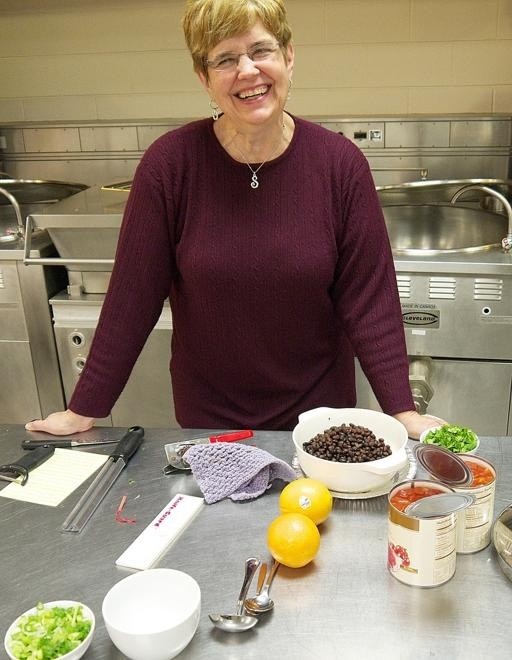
[219,116,292,189]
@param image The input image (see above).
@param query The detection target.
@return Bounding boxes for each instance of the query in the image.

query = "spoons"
[244,556,281,612]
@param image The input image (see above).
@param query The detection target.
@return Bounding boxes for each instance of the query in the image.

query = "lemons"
[267,513,321,569]
[280,478,331,525]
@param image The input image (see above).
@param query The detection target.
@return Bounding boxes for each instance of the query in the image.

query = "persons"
[23,0,450,444]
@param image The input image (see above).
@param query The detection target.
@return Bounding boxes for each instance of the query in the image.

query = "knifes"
[20,439,121,450]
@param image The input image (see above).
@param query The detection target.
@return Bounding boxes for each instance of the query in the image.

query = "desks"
[0,423,512,660]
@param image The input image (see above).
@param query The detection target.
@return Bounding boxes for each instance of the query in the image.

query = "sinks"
[381,204,508,253]
[0,203,55,236]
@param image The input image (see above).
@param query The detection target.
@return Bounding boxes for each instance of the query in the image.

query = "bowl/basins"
[102,568,202,660]
[4,600,96,660]
[419,425,480,455]
[292,405,409,493]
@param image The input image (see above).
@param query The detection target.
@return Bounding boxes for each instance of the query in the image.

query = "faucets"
[450,184,512,238]
[0,188,23,226]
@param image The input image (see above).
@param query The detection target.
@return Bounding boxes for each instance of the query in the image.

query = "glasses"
[204,39,284,70]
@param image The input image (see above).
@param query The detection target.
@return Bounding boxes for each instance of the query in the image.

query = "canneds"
[387,444,497,589]
